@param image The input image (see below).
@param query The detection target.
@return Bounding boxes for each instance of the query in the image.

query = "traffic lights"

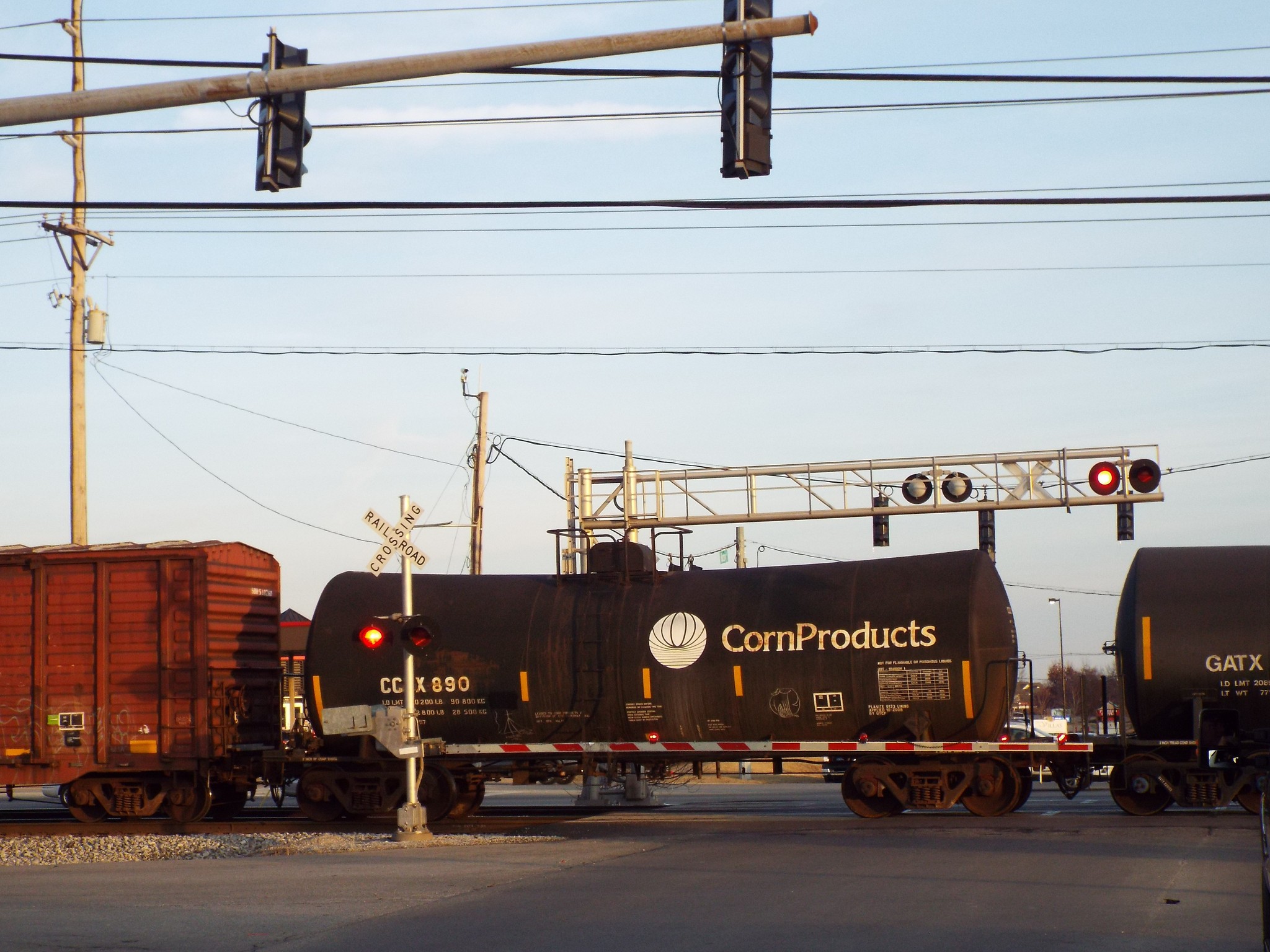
[1089,460,1162,495]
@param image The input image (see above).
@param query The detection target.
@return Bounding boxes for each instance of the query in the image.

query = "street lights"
[1048,598,1066,719]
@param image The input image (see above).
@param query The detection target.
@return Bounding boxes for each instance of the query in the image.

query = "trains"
[0,544,1270,831]
